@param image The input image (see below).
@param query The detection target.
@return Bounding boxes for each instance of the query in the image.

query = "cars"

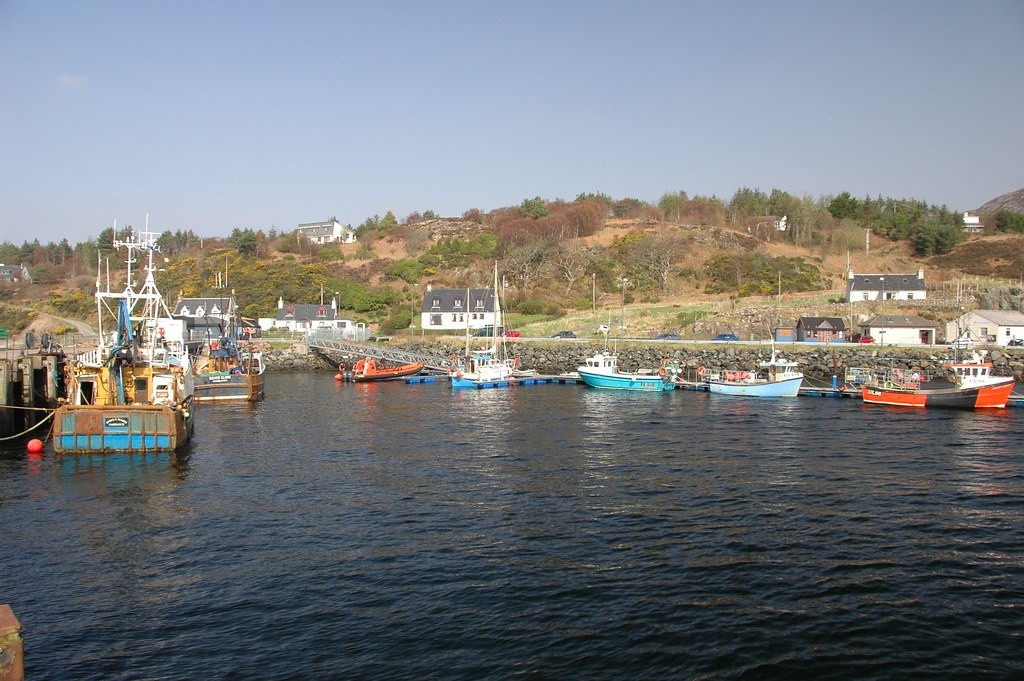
[1008,340,1024,346]
[711,334,738,341]
[653,332,681,340]
[951,338,972,345]
[497,331,521,337]
[857,336,876,343]
[550,331,576,338]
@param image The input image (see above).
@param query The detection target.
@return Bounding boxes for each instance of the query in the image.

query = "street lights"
[592,271,596,317]
[777,270,782,330]
[918,267,924,347]
[325,292,341,320]
[277,297,288,327]
[847,269,855,342]
[409,282,418,329]
[501,273,508,339]
[879,278,884,347]
[621,278,628,340]
[214,269,223,335]
[865,228,871,259]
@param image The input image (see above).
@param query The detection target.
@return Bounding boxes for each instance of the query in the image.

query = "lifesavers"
[698,366,706,376]
[883,380,893,390]
[659,367,667,376]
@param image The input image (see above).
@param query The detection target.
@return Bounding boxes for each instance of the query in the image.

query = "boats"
[308,344,425,383]
[578,323,677,392]
[861,380,1013,409]
[709,371,804,398]
[941,363,993,376]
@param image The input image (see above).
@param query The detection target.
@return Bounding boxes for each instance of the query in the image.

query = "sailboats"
[452,260,543,387]
[96,215,195,399]
[172,275,266,408]
[52,211,198,457]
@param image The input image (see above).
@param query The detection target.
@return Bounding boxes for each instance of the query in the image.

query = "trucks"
[471,327,503,338]
